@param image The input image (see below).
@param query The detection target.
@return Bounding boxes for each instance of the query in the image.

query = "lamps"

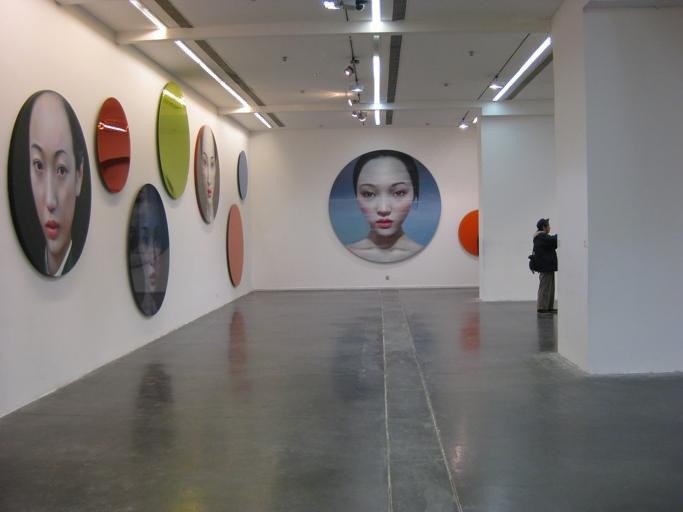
[458,32,530,129]
[325,0,367,126]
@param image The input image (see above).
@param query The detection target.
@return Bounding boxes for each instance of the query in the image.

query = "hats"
[537,218,549,232]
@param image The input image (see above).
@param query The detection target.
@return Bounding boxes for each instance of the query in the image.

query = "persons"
[529,217,558,312]
[346,150,424,263]
[138,187,165,315]
[200,125,217,222]
[28,91,85,277]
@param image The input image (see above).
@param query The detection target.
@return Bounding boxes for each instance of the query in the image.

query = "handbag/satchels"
[528,255,541,272]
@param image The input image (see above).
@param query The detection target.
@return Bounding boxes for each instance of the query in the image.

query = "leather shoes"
[537,308,557,313]
